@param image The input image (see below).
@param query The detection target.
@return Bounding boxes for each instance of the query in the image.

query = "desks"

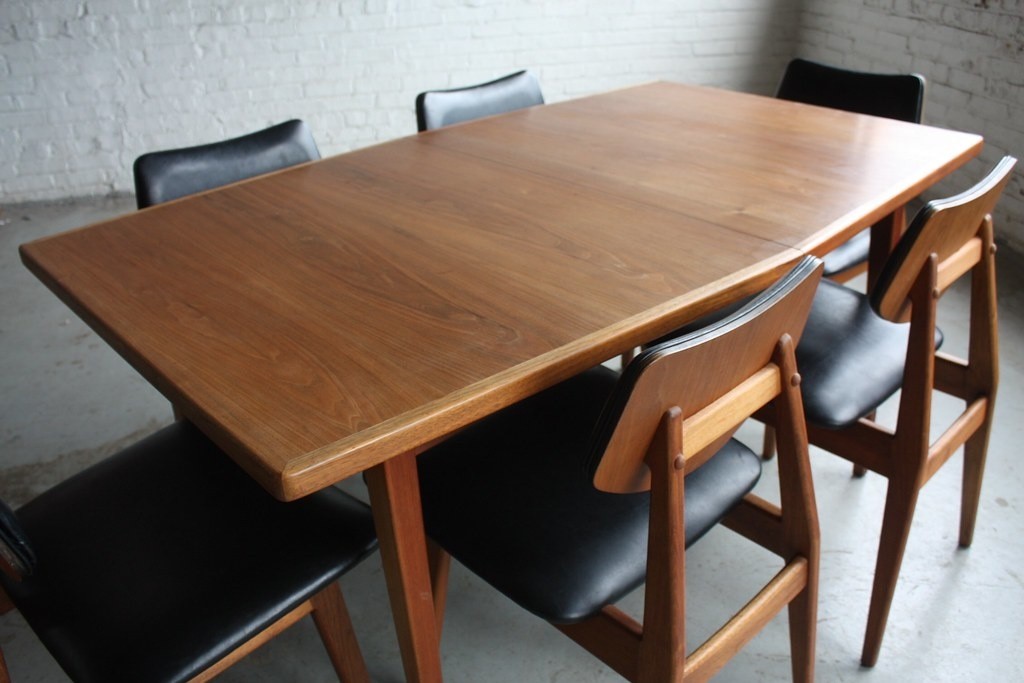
[19,82,984,683]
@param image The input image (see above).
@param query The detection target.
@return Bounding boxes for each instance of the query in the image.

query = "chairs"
[776,58,927,286]
[416,68,547,131]
[643,156,1017,669]
[0,418,381,683]
[362,255,824,683]
[132,118,322,213]
